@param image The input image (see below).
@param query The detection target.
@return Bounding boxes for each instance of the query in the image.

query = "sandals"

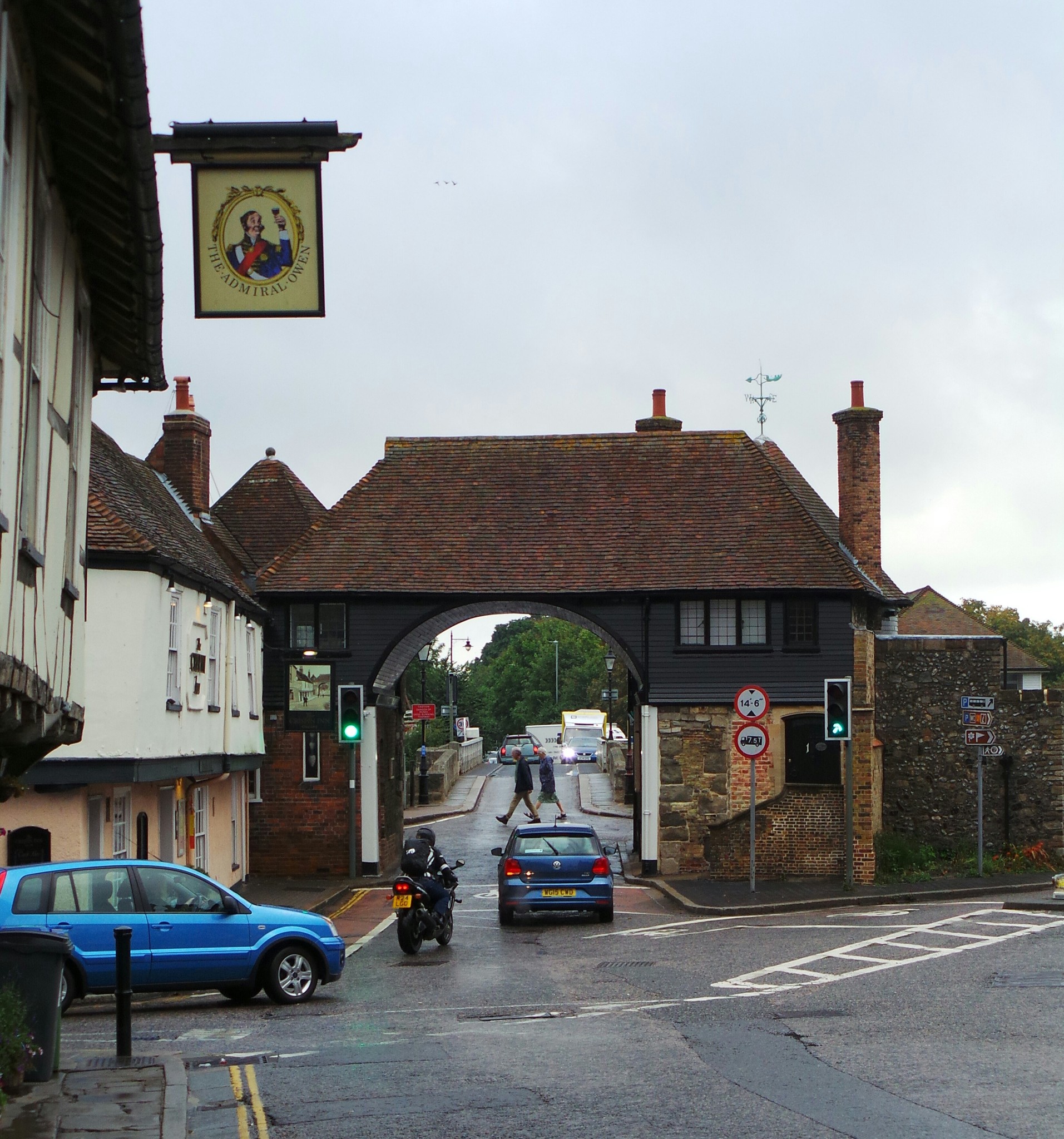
[556,813,567,820]
[523,812,533,820]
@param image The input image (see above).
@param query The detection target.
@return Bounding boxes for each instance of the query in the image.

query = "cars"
[559,737,602,764]
[490,822,615,925]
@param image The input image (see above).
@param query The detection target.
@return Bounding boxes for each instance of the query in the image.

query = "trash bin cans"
[0,930,75,1082]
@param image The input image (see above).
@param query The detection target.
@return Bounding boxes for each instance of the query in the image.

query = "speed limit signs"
[458,718,468,730]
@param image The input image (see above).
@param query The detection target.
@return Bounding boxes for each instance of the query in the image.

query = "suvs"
[483,733,541,765]
[0,857,349,1004]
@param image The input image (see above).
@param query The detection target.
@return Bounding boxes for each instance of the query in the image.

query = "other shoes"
[431,911,443,924]
[495,815,508,825]
[528,818,541,824]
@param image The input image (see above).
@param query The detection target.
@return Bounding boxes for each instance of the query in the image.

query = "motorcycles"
[386,860,465,952]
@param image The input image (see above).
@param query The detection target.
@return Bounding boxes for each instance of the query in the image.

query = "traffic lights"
[337,685,364,743]
[823,679,852,740]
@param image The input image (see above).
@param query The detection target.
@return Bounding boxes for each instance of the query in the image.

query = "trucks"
[525,709,626,764]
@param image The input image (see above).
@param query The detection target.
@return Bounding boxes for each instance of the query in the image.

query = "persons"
[496,747,541,824]
[523,747,567,819]
[148,876,195,912]
[403,827,457,925]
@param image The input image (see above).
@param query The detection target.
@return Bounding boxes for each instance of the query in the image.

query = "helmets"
[416,827,436,846]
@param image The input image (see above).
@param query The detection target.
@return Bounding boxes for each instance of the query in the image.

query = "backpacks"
[400,838,431,876]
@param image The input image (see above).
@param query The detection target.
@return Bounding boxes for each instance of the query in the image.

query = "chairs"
[117,879,135,912]
[96,880,116,912]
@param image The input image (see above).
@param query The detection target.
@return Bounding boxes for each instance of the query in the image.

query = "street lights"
[548,640,558,703]
[450,630,474,672]
[417,642,433,805]
[601,646,617,740]
